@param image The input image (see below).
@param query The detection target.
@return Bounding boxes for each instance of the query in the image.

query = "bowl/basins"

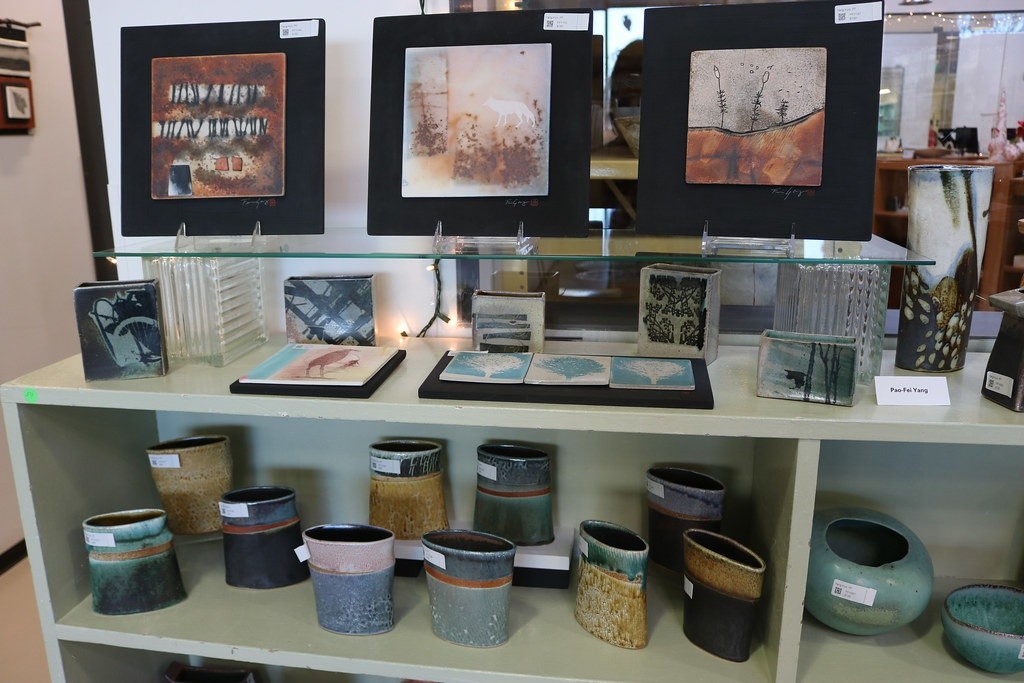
[940,582,1024,674]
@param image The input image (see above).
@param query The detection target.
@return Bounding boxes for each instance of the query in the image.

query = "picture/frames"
[634,1,886,241]
[366,6,593,237]
[120,18,327,237]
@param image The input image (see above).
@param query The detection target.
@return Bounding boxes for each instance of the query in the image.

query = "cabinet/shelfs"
[875,154,1024,312]
[0,236,1024,683]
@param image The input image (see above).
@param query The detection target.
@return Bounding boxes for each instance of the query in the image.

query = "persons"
[602,39,642,231]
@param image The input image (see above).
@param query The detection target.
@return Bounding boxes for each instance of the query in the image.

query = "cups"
[895,163,994,373]
